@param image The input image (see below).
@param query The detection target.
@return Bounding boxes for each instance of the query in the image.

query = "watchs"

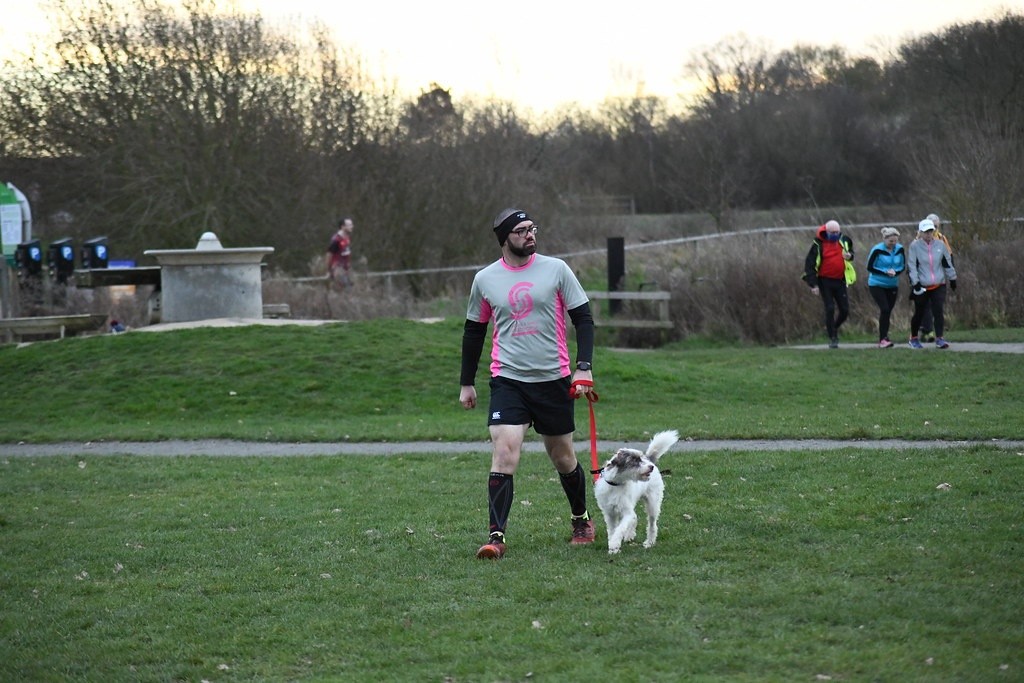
[576,363,592,371]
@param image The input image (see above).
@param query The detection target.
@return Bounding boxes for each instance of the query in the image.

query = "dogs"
[593,429,681,555]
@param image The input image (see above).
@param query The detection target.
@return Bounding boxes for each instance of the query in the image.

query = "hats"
[918,220,935,232]
[926,213,941,228]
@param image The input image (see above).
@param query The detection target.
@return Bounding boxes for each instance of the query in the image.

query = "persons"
[801,220,855,348]
[908,213,957,349]
[327,218,354,275]
[459,209,595,561]
[866,226,906,349]
[111,320,125,331]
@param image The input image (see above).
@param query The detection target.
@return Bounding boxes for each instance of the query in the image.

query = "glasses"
[511,226,538,238]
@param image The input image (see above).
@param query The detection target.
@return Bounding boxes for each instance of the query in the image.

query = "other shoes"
[833,328,838,337]
[926,332,935,343]
[829,338,838,348]
[919,334,925,342]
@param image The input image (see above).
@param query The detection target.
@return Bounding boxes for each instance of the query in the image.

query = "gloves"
[950,280,957,291]
[913,288,927,295]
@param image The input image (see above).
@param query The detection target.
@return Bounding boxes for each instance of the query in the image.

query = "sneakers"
[936,337,949,348]
[909,338,922,349]
[477,533,506,559]
[879,337,893,347]
[570,512,595,545]
